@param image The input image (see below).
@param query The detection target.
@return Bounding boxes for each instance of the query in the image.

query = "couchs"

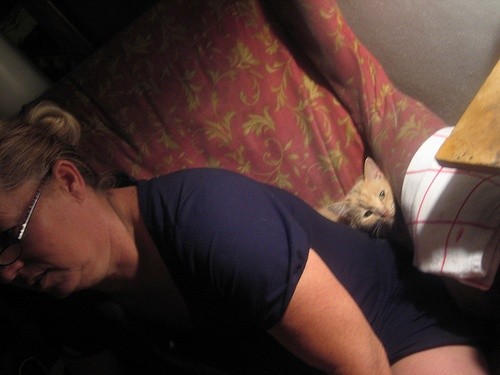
[0,0,499,353]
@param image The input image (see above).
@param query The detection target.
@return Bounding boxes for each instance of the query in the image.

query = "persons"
[1,98,494,375]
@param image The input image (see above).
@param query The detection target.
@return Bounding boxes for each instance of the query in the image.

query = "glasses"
[0,167,53,267]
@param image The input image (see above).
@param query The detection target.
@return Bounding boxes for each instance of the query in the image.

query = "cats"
[318,156,395,235]
